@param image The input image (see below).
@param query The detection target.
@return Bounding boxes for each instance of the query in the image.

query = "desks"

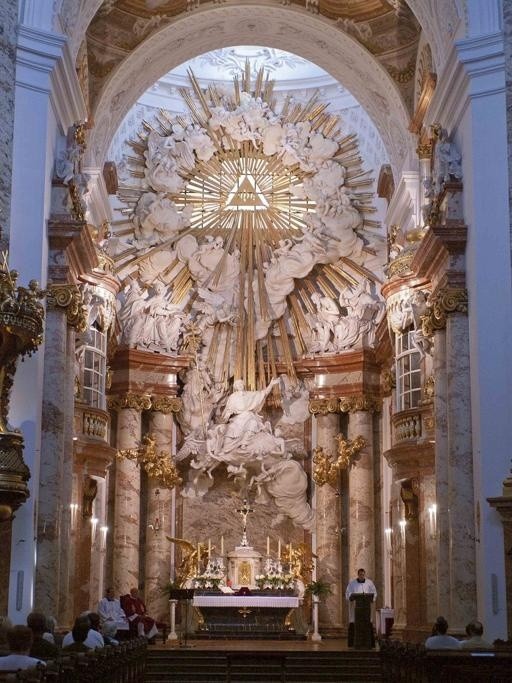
[191,595,303,640]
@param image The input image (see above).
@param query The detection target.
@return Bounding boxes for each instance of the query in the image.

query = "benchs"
[377,636,511,683]
[1,633,149,683]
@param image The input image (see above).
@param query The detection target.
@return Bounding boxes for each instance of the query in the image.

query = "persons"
[344,568,378,648]
[221,376,277,455]
[118,93,385,357]
[0,586,159,670]
[425,614,495,649]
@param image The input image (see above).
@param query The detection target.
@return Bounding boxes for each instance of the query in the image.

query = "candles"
[221,535,224,553]
[288,541,292,561]
[266,535,270,554]
[208,538,211,557]
[277,538,281,558]
[197,542,200,559]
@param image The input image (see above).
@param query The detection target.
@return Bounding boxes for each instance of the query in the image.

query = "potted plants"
[304,576,336,602]
[158,581,182,599]
